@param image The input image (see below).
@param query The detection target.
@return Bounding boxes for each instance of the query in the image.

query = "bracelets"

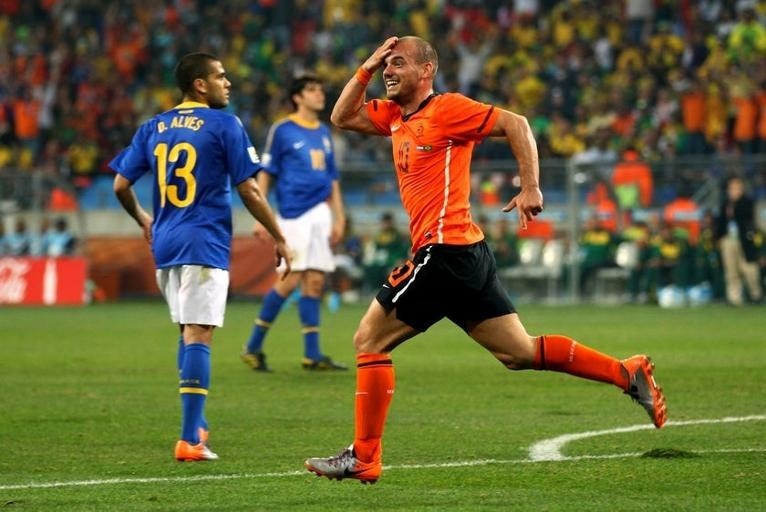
[352,66,374,87]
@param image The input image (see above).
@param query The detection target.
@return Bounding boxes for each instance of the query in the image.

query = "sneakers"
[301,354,348,371]
[619,351,669,430]
[302,443,384,485]
[239,343,274,373]
[175,427,220,462]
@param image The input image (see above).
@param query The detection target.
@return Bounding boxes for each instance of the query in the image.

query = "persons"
[305,36,668,485]
[108,52,290,460]
[239,72,349,375]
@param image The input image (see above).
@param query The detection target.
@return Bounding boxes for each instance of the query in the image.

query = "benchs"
[494,259,634,310]
